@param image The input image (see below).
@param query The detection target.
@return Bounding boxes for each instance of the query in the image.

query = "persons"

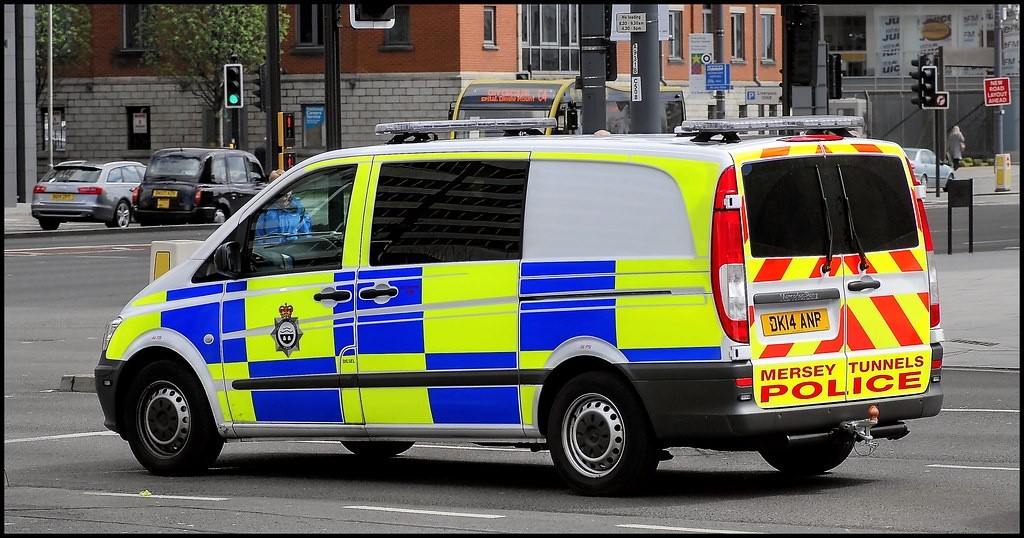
[947,126,965,171]
[254,169,312,240]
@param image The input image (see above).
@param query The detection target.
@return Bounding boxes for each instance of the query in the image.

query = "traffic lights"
[224,64,243,109]
[908,55,922,108]
[920,65,937,110]
[279,152,296,173]
[277,112,296,146]
[515,73,530,81]
[252,62,267,112]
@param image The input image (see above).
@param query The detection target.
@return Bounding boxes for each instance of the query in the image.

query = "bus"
[447,79,684,138]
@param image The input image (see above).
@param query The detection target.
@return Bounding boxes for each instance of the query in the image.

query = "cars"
[30,161,154,229]
[131,146,272,226]
[903,148,956,193]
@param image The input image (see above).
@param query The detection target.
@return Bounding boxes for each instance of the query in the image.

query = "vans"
[85,116,944,500]
